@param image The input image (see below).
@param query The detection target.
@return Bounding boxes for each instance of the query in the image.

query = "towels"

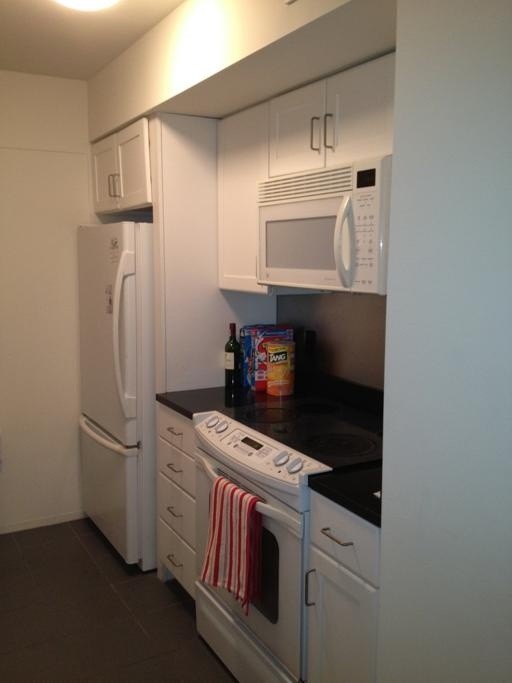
[199,475,263,617]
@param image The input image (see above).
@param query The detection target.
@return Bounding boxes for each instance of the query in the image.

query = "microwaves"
[255,156,389,297]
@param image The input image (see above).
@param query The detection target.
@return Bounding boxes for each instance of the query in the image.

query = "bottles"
[224,323,241,388]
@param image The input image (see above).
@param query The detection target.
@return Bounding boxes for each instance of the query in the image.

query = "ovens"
[195,448,305,683]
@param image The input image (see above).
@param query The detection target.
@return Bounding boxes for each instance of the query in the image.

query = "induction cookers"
[194,402,383,496]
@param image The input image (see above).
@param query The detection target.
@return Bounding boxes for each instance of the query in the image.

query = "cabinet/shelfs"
[217,101,332,297]
[155,395,195,606]
[305,476,381,683]
[91,116,152,214]
[268,51,391,177]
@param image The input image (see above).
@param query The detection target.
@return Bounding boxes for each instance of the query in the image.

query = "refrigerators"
[78,222,155,571]
